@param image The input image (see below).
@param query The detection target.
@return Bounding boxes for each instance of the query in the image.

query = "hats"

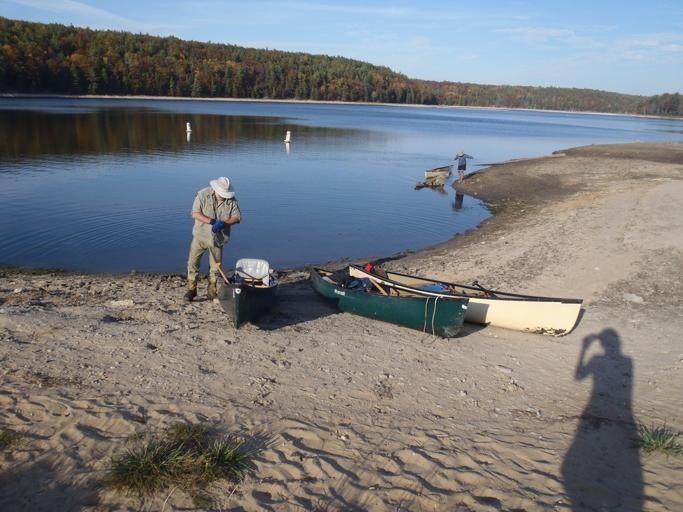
[210,176,235,199]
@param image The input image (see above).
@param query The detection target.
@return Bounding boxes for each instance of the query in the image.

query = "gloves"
[210,219,225,233]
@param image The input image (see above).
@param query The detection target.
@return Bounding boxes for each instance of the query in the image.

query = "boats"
[310,267,470,338]
[349,263,584,338]
[424,165,455,180]
[217,259,279,330]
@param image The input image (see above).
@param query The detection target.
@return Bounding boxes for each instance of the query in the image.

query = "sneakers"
[183,288,197,301]
[207,290,218,299]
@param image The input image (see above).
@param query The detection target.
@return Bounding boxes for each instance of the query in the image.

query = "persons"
[448,190,469,213]
[454,149,474,184]
[180,176,241,302]
